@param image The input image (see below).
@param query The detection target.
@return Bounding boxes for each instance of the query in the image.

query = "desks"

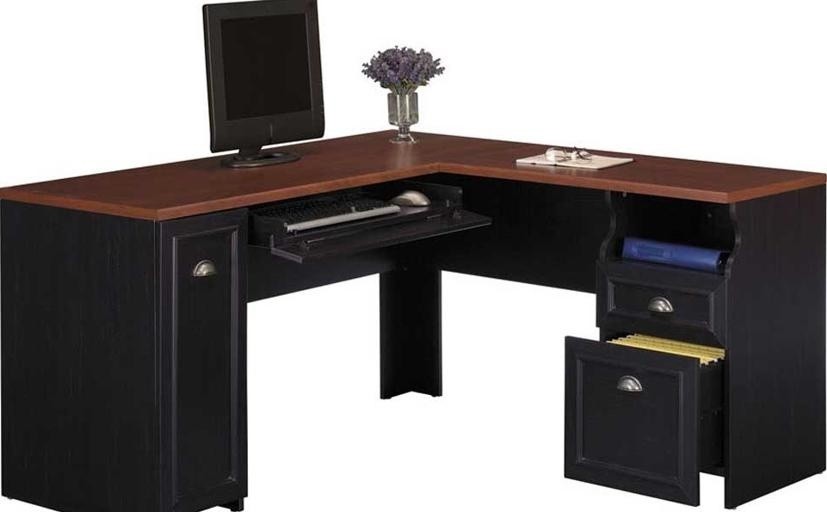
[1,128,827,512]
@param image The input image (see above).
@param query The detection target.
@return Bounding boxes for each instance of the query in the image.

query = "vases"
[387,92,419,144]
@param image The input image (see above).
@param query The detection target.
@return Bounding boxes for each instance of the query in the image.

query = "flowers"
[361,45,446,124]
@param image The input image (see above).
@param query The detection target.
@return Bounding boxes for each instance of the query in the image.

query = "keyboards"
[255,192,401,233]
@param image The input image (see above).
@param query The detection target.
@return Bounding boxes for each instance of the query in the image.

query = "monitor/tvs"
[202,1,324,169]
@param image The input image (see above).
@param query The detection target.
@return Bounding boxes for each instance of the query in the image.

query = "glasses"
[545,146,592,165]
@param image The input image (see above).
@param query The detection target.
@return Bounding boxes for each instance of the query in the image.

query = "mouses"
[389,189,434,206]
